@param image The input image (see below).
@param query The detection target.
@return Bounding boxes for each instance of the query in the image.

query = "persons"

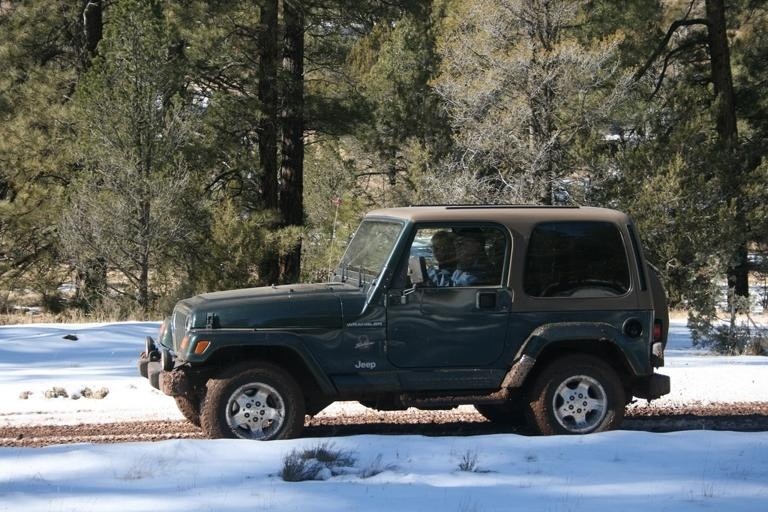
[423,229,459,287]
[442,226,502,287]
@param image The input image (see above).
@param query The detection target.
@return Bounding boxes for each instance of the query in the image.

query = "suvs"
[134,203,670,442]
